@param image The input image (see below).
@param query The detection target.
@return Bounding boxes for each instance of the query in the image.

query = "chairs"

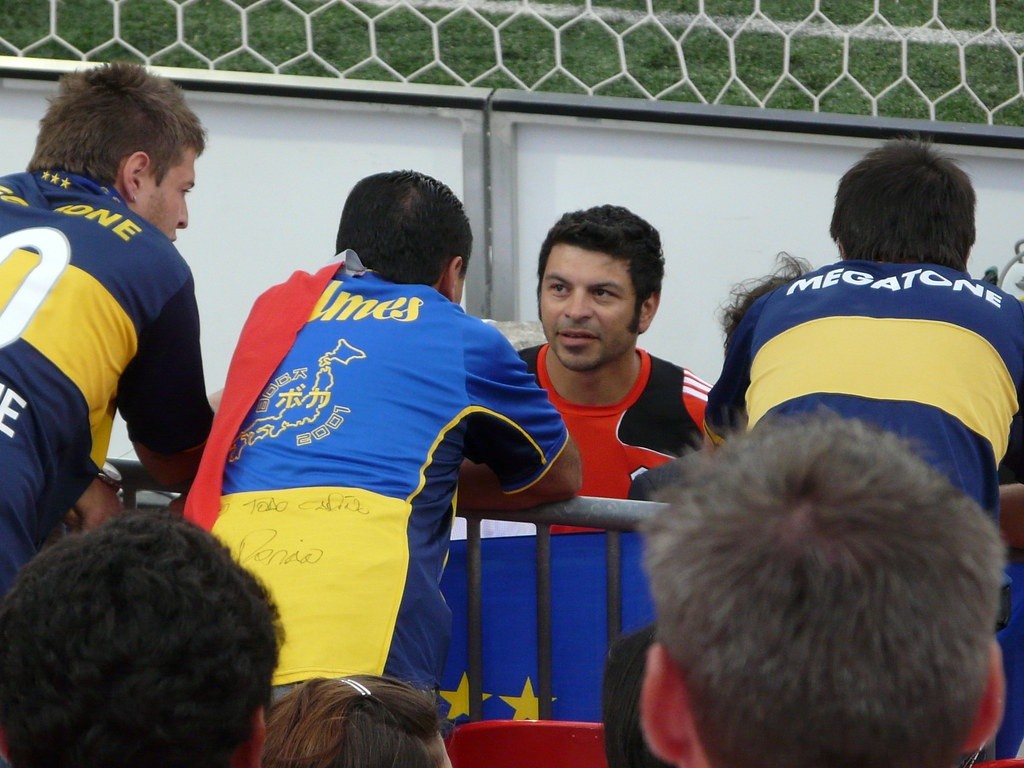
[449,721,610,768]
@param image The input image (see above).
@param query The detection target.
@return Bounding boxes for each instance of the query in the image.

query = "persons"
[702,134,1024,759]
[0,509,286,768]
[514,204,716,523]
[180,170,584,695]
[640,403,1005,768]
[2,56,224,589]
[262,674,454,768]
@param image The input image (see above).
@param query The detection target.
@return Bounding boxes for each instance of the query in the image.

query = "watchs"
[96,460,123,495]
